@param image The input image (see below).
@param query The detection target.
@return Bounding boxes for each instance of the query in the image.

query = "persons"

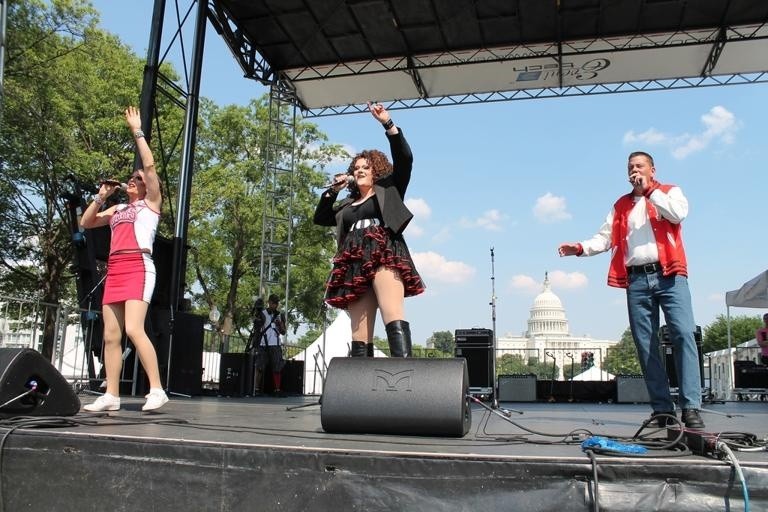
[312,100,428,360]
[251,292,286,398]
[755,313,768,366]
[556,149,709,431]
[80,105,172,414]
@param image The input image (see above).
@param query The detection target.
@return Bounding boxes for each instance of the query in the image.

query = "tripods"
[285,305,328,412]
[475,248,524,418]
[145,302,193,398]
[75,273,108,396]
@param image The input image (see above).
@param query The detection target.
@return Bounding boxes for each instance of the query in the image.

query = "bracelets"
[90,194,105,208]
[382,119,394,131]
[326,187,339,197]
[132,130,144,139]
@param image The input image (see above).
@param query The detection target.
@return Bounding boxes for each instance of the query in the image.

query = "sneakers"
[83,396,120,412]
[142,390,168,411]
[642,411,675,428]
[681,409,704,428]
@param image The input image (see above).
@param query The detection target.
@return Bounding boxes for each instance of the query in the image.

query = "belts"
[626,263,660,274]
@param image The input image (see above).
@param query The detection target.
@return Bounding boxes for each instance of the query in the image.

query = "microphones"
[630,169,638,185]
[98,178,130,191]
[324,174,355,189]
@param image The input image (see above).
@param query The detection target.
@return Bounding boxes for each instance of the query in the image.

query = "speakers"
[659,342,706,389]
[0,348,80,417]
[321,358,470,438]
[454,346,495,396]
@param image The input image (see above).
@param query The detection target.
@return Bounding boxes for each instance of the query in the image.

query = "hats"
[269,294,279,301]
